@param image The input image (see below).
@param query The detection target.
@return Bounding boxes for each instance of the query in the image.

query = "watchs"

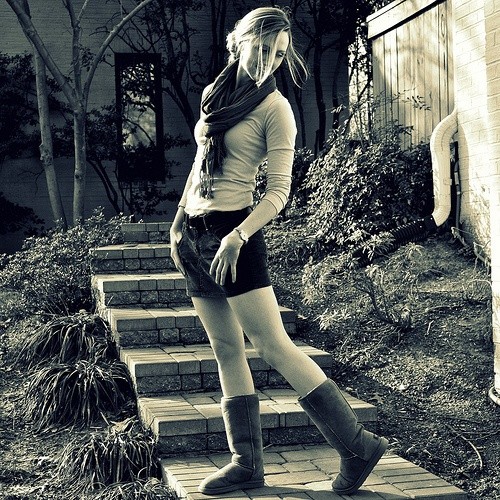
[234,227,250,244]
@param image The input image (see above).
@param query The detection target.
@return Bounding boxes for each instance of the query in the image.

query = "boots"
[297,378,389,494]
[199,393,264,494]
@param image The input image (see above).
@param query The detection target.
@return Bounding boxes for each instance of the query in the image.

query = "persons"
[168,7,390,495]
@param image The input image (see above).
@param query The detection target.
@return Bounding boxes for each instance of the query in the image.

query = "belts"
[184,206,252,231]
